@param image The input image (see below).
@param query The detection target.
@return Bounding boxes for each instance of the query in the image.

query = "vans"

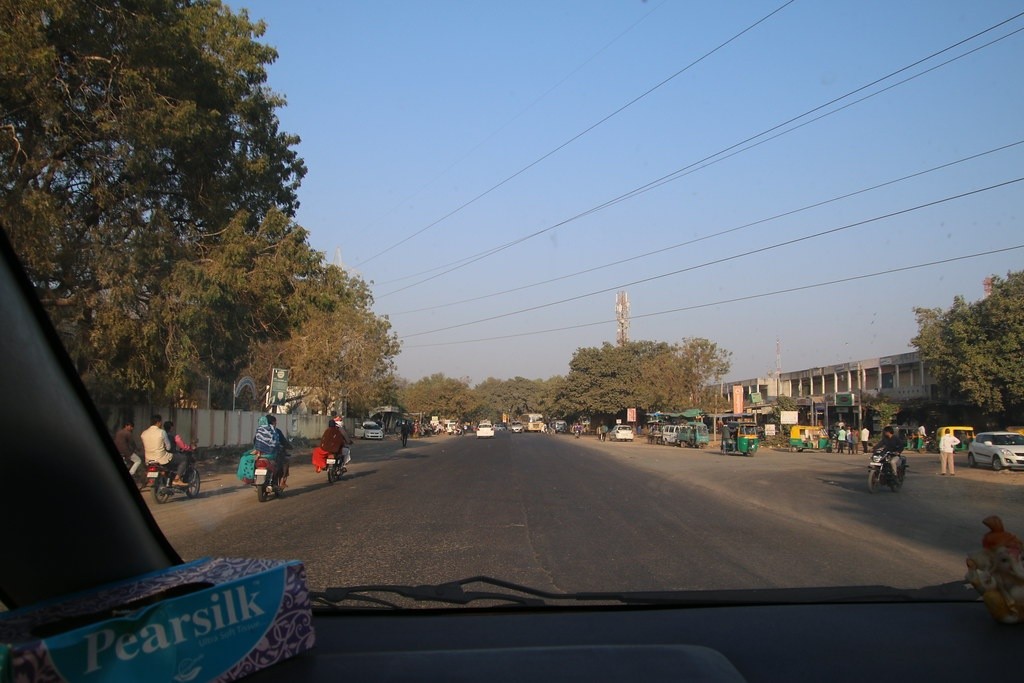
[663,425,692,446]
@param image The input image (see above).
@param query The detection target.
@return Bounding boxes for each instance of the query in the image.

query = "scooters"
[575,430,579,438]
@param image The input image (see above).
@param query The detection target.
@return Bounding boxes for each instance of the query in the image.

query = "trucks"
[552,420,567,433]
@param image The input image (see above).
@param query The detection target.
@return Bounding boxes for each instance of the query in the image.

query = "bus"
[521,414,543,433]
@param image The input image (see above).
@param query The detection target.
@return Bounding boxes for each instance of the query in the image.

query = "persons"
[638,424,661,444]
[332,417,353,472]
[114,415,294,490]
[939,429,961,476]
[321,419,347,464]
[722,423,739,455]
[394,420,473,448]
[546,419,608,442]
[375,418,382,428]
[829,419,870,455]
[901,417,928,452]
[869,426,903,480]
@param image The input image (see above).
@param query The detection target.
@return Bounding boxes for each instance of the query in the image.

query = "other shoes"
[280,483,289,488]
[172,479,188,486]
[342,465,347,472]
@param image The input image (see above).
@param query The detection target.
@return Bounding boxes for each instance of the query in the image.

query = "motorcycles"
[326,434,355,483]
[146,438,201,505]
[866,443,909,494]
[893,426,977,453]
[790,425,833,454]
[720,422,759,456]
[254,438,294,502]
[678,422,710,449]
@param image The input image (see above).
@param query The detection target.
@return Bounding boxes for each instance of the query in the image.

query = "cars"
[355,421,384,440]
[493,424,506,431]
[967,432,1024,472]
[447,423,456,435]
[511,421,524,433]
[476,423,494,439]
[609,425,634,442]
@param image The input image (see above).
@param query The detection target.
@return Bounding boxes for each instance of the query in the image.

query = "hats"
[334,416,343,426]
